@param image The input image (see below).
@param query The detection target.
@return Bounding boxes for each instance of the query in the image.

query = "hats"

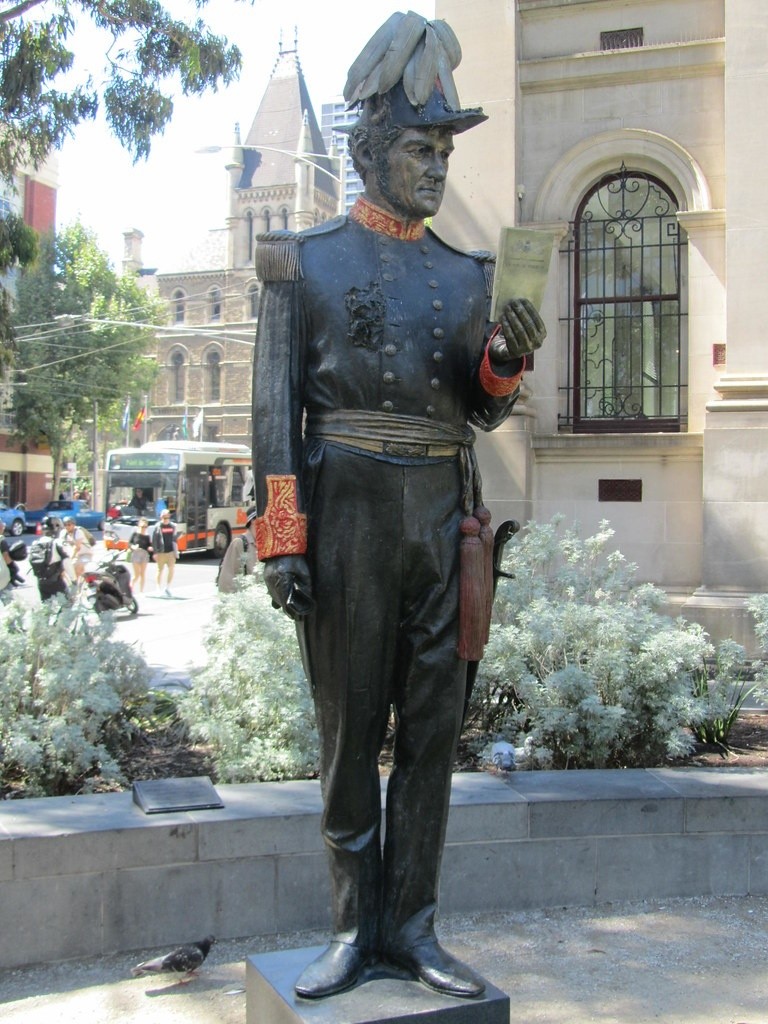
[160,508,171,518]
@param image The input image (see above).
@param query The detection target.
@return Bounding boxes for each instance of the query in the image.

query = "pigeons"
[130,934,218,987]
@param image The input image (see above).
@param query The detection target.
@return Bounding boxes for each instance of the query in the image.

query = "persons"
[29,515,92,628]
[216,506,258,604]
[0,517,25,607]
[152,509,177,597]
[59,486,150,516]
[129,518,153,593]
[252,11,547,998]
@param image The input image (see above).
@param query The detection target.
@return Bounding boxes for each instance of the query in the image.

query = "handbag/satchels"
[124,549,133,564]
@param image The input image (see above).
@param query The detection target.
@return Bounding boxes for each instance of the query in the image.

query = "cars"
[25,498,106,532]
[0,500,24,537]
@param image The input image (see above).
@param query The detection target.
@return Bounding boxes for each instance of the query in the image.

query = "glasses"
[139,525,149,528]
[64,523,73,525]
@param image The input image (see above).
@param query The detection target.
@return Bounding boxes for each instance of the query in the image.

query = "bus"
[99,434,259,560]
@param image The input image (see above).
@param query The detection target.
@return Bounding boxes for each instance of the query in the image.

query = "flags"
[181,404,204,440]
[122,393,147,431]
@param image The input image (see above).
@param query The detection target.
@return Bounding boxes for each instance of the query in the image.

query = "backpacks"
[28,543,54,576]
